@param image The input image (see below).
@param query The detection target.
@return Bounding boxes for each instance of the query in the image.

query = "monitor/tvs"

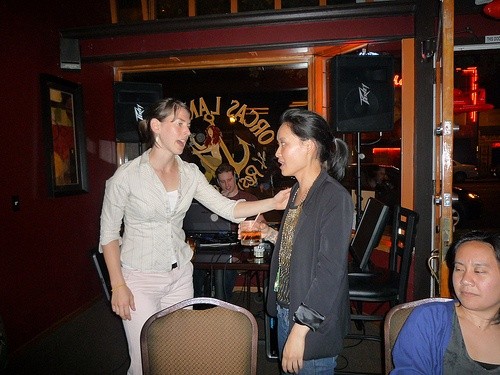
[348,197,390,276]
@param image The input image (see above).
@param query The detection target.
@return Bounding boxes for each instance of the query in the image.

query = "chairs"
[384,297,454,375]
[140,296,260,375]
[343,207,418,375]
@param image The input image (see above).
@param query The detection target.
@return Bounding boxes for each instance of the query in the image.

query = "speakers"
[330,55,394,132]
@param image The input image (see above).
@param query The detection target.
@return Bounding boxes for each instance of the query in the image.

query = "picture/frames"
[38,72,89,198]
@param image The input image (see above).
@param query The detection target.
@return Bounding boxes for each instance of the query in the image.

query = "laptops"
[182,203,236,234]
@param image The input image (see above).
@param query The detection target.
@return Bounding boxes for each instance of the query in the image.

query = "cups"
[185,238,196,253]
[254,247,266,257]
[240,220,262,246]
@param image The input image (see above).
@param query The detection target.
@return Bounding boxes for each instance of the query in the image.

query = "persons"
[238,107,354,375]
[389,232,500,375]
[361,167,386,192]
[100,99,292,375]
[209,164,267,301]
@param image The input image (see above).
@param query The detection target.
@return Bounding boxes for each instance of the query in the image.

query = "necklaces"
[292,188,311,204]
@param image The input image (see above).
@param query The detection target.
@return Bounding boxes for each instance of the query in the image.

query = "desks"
[191,238,273,299]
